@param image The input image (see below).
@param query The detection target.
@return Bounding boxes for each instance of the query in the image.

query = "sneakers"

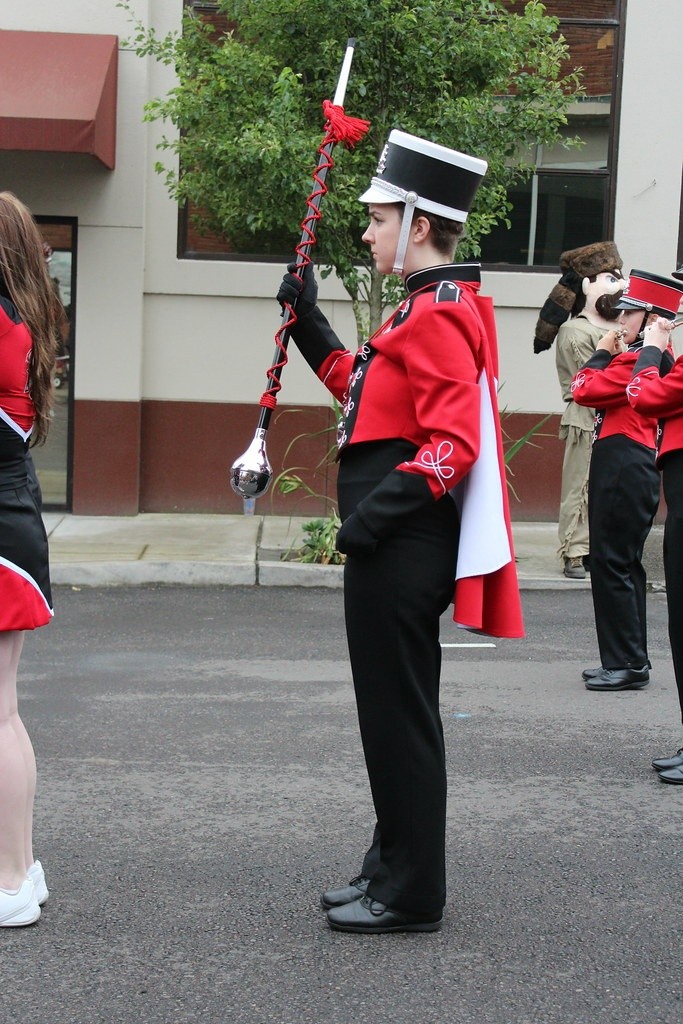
[564,555,587,578]
[0,859,51,927]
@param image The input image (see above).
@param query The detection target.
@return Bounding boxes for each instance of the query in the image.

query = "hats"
[533,240,623,354]
[357,129,488,274]
[610,270,683,347]
[671,266,683,281]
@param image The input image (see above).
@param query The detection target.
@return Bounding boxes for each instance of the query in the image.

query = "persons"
[0,193,62,931]
[623,317,683,792]
[533,239,632,579]
[275,129,484,935]
[569,268,683,693]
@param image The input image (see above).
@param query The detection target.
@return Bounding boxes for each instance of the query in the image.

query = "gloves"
[335,512,376,557]
[277,261,318,322]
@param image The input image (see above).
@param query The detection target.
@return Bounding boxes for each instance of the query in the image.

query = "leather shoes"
[581,665,650,691]
[320,876,444,933]
[651,749,683,785]
